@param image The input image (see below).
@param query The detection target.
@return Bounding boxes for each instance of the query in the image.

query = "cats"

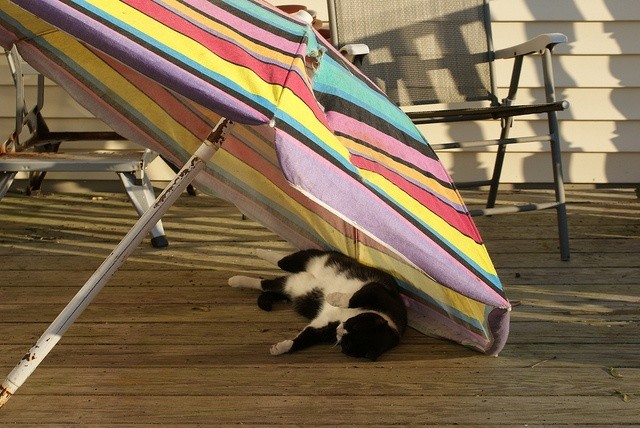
[227,248,409,362]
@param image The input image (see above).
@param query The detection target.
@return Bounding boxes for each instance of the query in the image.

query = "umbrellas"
[0,0,513,418]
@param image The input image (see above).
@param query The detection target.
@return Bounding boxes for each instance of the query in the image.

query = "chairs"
[326,0,572,260]
